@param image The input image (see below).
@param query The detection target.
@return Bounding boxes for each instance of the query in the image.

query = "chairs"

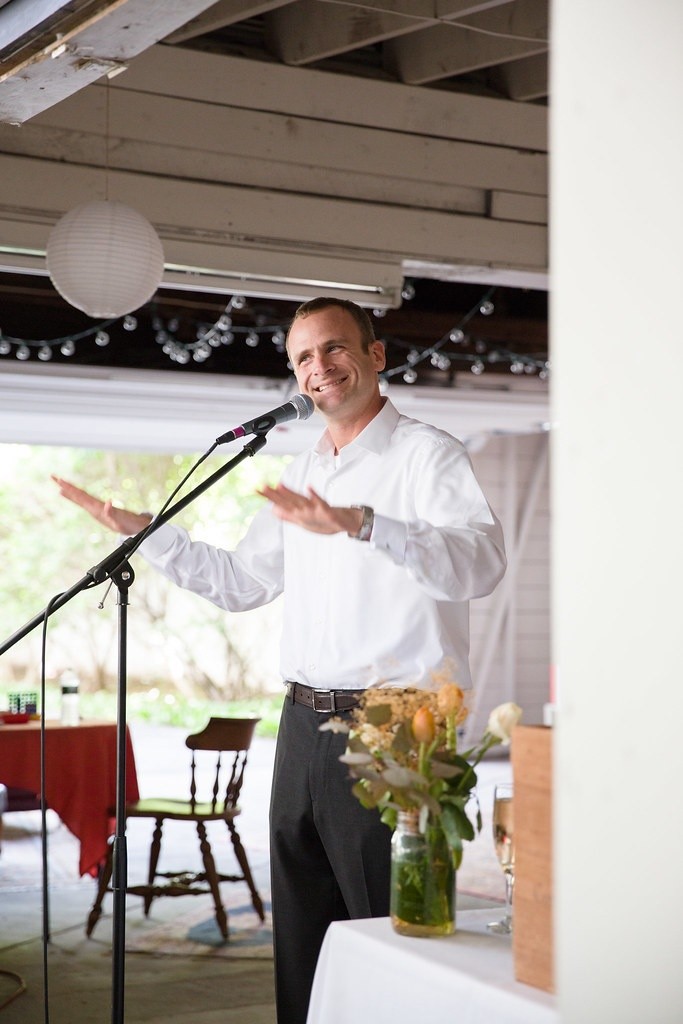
[84,719,266,939]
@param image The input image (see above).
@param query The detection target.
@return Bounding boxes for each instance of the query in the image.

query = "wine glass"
[487,782,514,936]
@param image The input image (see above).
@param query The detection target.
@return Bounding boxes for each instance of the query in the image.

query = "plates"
[29,715,41,719]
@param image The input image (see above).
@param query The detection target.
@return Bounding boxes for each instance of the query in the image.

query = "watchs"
[348,503,374,541]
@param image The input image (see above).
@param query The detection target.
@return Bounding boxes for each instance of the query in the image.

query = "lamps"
[0,212,404,308]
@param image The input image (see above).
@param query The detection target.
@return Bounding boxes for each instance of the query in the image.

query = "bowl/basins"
[1,714,29,723]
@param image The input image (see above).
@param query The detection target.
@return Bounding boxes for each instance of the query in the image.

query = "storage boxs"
[511,724,553,991]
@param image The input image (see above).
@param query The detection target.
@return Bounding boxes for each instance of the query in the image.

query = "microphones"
[215,393,315,445]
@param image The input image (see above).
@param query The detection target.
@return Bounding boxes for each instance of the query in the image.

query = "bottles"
[60,659,79,726]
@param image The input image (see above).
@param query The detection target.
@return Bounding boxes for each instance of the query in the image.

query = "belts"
[283,681,369,716]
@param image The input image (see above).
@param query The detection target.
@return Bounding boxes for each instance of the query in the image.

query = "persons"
[50,295,507,1024]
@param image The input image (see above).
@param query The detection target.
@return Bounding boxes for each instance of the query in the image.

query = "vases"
[390,810,457,937]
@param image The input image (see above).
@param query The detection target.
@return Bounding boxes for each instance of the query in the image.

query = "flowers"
[317,683,519,837]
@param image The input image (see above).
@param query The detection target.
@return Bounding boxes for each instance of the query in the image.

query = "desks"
[0,718,141,874]
[301,906,560,1024]
